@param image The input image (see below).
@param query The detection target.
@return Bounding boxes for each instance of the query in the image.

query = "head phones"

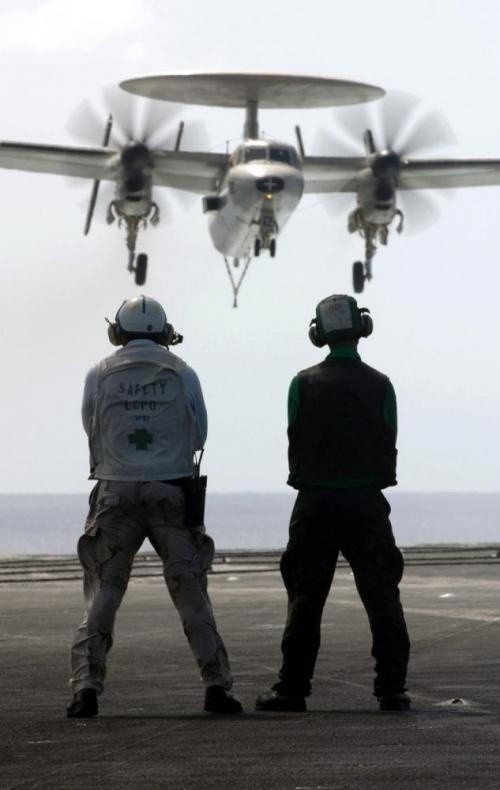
[309,294,374,345]
[108,297,176,346]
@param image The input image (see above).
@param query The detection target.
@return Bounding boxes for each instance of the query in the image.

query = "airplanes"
[0,70,500,305]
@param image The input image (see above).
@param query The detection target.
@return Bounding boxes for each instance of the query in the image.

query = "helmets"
[116,294,168,336]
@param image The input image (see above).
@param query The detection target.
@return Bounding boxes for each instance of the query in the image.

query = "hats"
[315,294,361,339]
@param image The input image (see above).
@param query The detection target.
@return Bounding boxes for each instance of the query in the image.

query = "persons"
[66,295,245,717]
[254,294,412,714]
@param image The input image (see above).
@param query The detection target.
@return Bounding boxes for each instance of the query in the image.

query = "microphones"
[103,317,112,325]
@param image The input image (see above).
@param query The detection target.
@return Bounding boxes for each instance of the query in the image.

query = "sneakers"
[65,686,99,720]
[202,684,244,717]
[377,690,412,713]
[253,687,308,713]
